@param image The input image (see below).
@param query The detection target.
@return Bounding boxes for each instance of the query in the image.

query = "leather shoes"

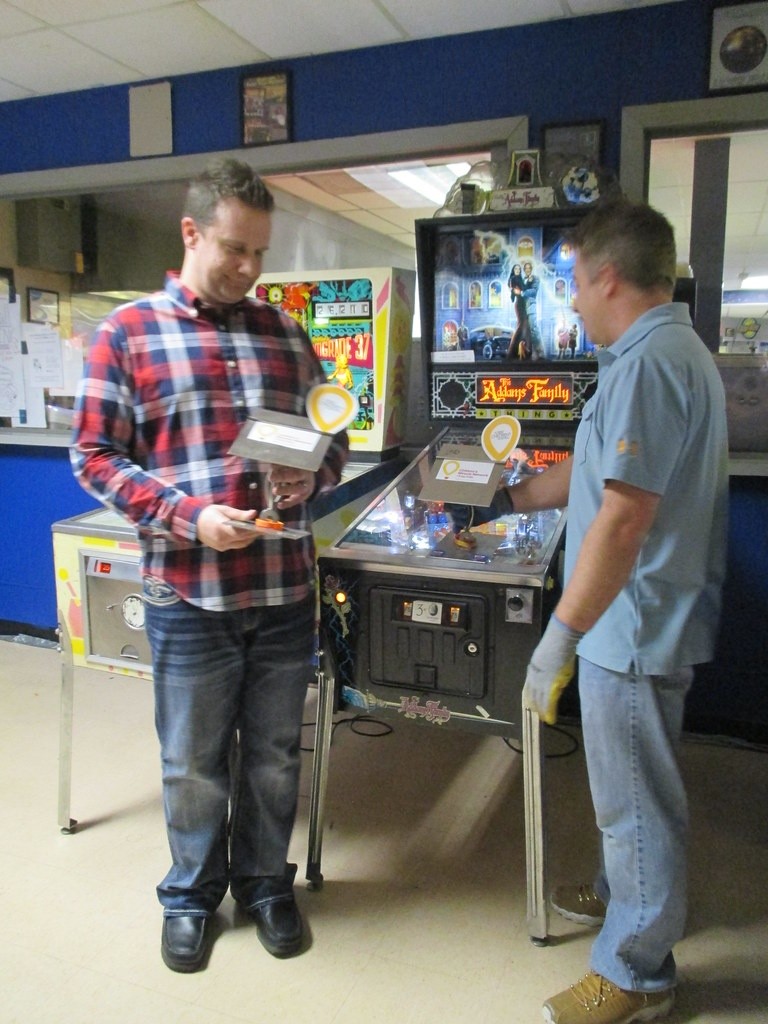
[252,896,303,956]
[161,917,209,972]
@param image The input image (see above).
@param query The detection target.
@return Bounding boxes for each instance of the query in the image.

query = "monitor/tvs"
[422,213,605,361]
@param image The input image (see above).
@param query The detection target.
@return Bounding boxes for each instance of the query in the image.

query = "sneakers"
[550,884,607,927]
[542,969,675,1024]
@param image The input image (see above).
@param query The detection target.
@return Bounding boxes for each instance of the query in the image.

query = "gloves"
[444,487,514,534]
[523,612,585,725]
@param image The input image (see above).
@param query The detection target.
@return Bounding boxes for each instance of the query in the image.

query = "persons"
[443,197,731,1024]
[70,162,349,973]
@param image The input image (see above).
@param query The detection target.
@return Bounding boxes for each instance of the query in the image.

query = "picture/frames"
[26,287,60,324]
